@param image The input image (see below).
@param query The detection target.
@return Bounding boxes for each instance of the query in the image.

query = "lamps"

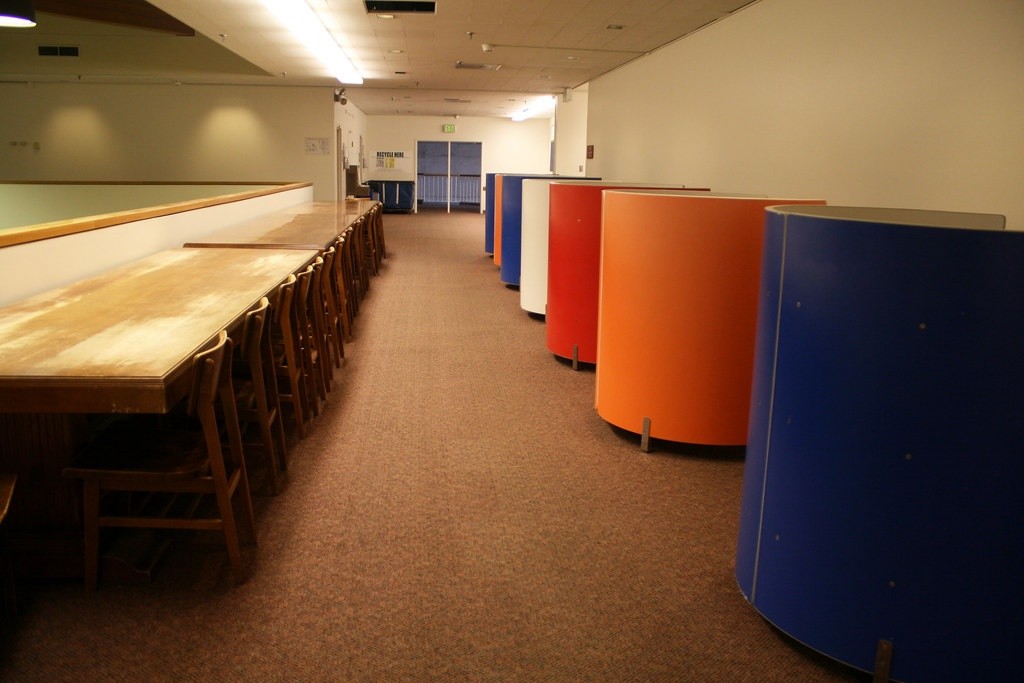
[0,0,38,28]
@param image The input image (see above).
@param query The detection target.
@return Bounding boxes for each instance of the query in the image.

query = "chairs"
[0,201,384,607]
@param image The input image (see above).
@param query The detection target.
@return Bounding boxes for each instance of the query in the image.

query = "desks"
[286,199,377,214]
[3,248,320,416]
[183,212,360,249]
